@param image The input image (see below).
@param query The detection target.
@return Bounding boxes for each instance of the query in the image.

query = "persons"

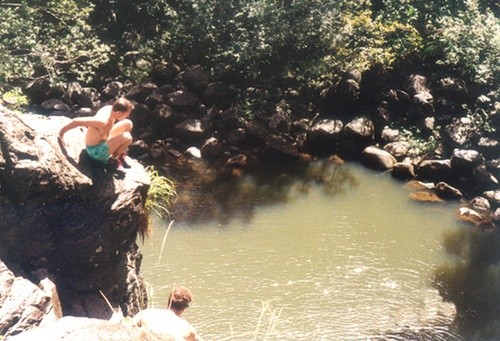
[130,288,201,341]
[57,97,135,166]
